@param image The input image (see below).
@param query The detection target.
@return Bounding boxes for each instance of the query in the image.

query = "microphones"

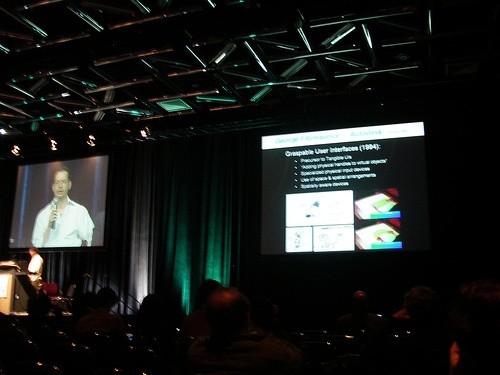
[52,198,58,229]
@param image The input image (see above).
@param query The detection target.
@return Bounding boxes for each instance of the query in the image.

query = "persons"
[27,247,44,295]
[0,278,500,375]
[31,168,94,247]
